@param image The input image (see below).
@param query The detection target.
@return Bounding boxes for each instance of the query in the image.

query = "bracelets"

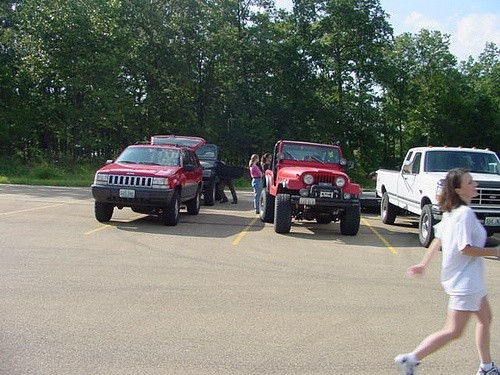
[496,247,500,256]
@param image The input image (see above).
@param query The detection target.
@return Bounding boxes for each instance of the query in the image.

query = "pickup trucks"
[375,146,500,247]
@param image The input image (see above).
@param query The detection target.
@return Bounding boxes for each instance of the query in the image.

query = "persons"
[395,167,500,375]
[215,153,272,214]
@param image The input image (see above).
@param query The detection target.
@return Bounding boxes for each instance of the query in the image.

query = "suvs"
[90,133,208,226]
[257,138,362,236]
[196,143,229,206]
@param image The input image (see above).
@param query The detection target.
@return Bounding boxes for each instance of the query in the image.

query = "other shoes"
[220,199,228,203]
[231,202,237,204]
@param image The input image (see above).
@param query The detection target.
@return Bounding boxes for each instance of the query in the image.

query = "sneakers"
[393,352,422,375]
[476,364,500,375]
